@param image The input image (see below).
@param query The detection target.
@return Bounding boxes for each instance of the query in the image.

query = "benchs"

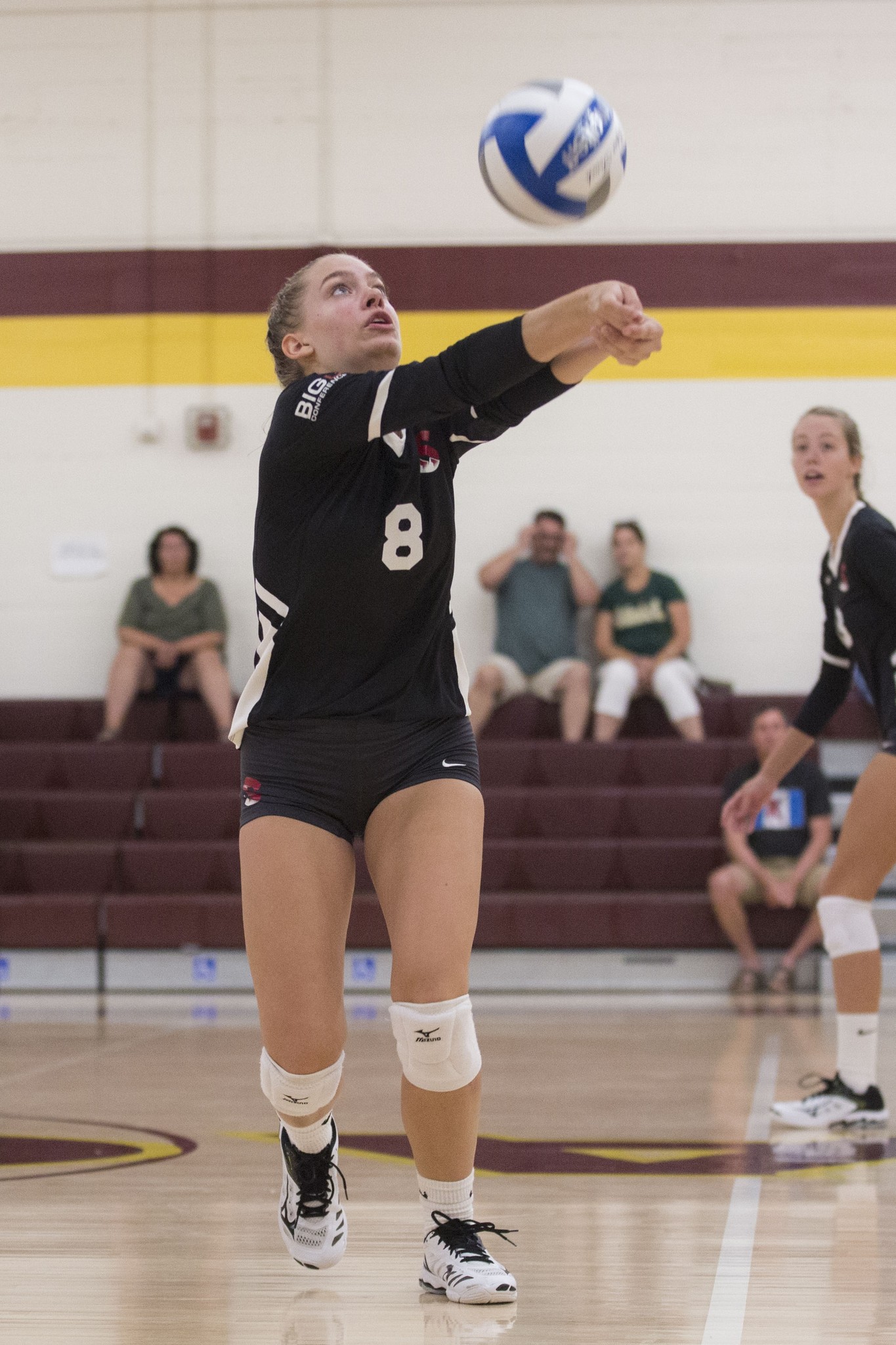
[0,696,896,950]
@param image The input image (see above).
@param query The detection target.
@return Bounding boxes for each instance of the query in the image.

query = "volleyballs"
[479,75,627,228]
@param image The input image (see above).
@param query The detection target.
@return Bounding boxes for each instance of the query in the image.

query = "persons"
[715,409,896,1125]
[229,252,665,1305]
[88,525,240,746]
[590,521,708,744]
[464,510,601,743]
[704,703,837,996]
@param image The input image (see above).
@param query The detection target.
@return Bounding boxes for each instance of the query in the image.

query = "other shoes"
[730,962,795,996]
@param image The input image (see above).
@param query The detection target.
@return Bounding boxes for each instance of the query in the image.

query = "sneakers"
[278,1113,348,1268]
[767,1072,887,1127]
[419,1211,518,1305]
